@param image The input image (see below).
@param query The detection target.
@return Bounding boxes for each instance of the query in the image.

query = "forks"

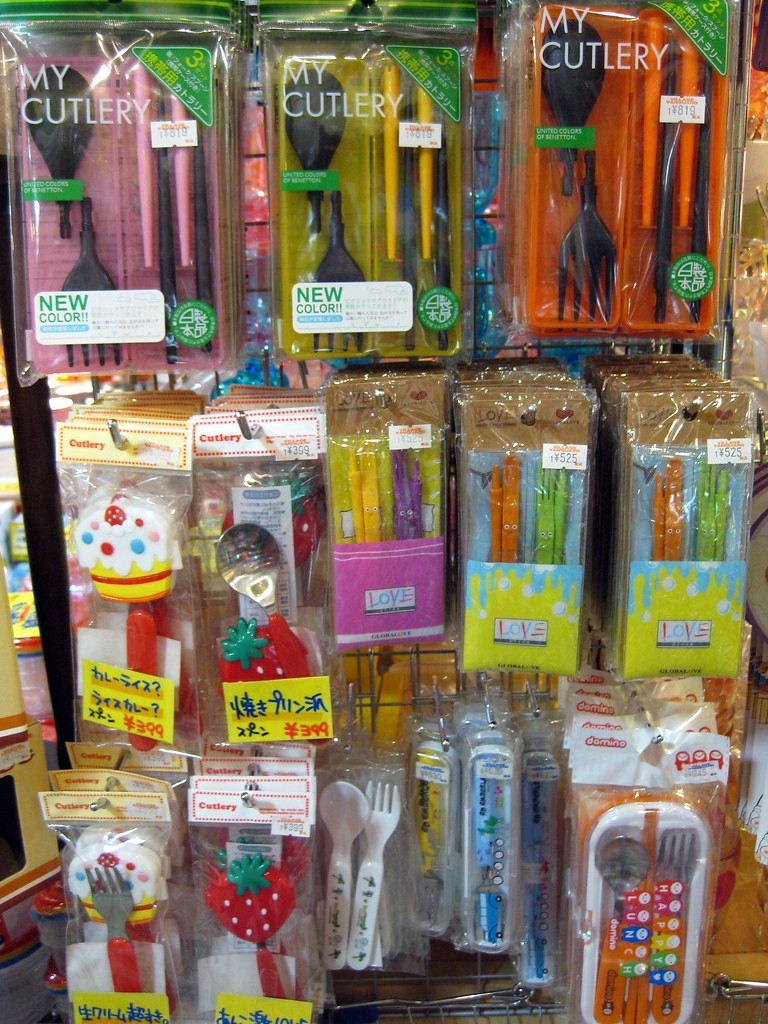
[59,197,122,367]
[347,780,402,970]
[652,833,695,1024]
[310,192,367,352]
[558,149,616,322]
[473,845,506,942]
[85,867,143,992]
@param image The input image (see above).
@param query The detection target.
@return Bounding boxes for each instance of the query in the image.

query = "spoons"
[594,837,648,1024]
[318,780,369,970]
[25,66,96,240]
[542,19,606,196]
[217,524,334,745]
[287,70,346,233]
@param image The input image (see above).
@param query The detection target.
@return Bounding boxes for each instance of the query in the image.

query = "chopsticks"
[653,41,712,323]
[400,87,451,351]
[156,86,214,365]
